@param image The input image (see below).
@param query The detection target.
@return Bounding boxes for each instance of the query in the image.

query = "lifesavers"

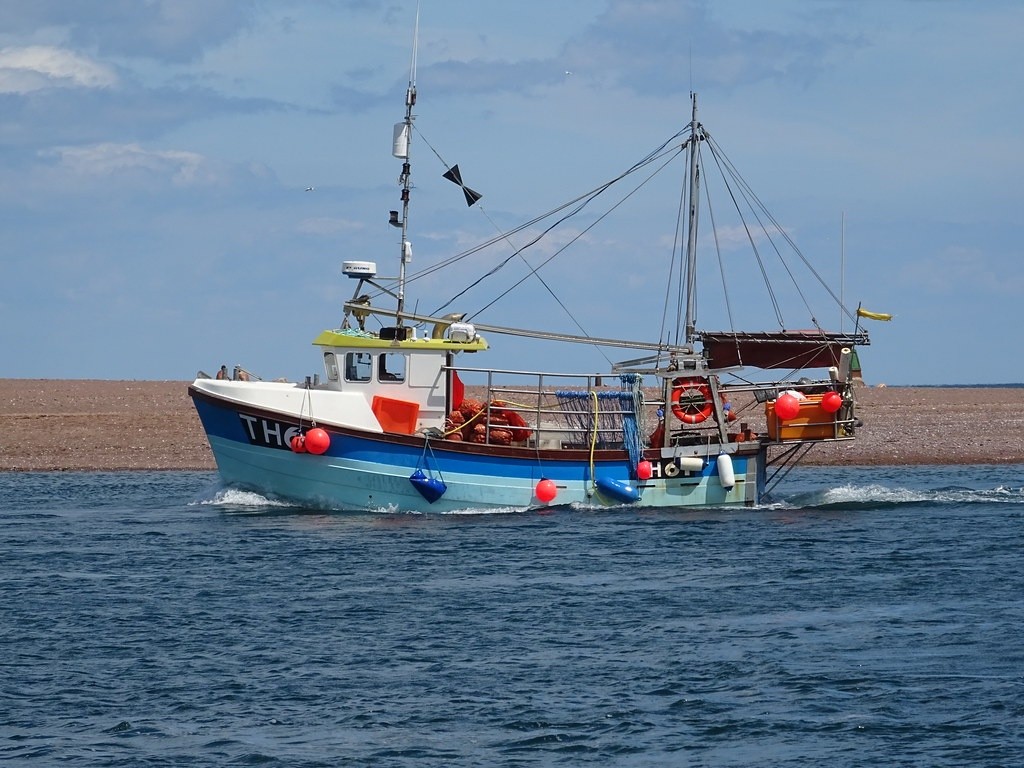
[671,382,714,424]
[671,375,710,414]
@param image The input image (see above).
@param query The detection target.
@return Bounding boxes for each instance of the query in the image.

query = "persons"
[216,365,226,380]
[239,372,249,381]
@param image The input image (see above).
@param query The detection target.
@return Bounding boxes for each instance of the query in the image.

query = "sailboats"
[186,1,872,517]
